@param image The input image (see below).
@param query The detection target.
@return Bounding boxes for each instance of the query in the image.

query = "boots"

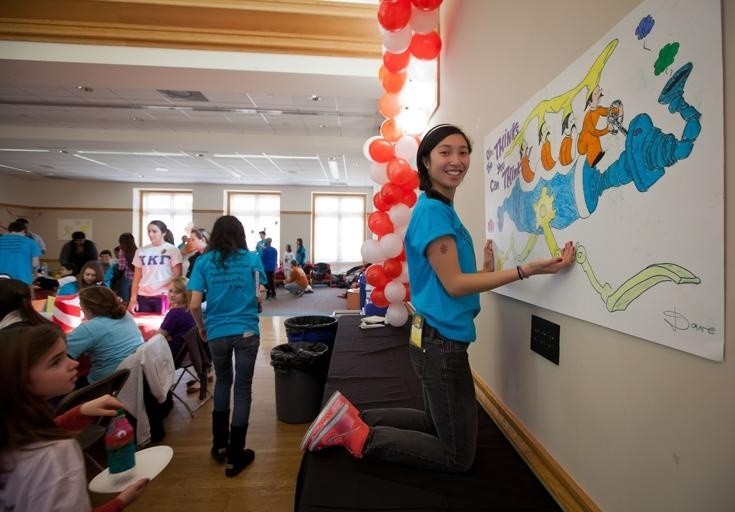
[212,410,228,461]
[226,422,254,476]
[300,390,370,459]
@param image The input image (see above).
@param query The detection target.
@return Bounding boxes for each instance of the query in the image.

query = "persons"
[0,319,151,512]
[297,122,578,475]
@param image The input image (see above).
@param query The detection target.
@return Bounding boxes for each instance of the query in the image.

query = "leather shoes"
[187,376,213,392]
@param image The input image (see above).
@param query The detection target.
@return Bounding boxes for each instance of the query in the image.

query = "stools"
[346,289,360,309]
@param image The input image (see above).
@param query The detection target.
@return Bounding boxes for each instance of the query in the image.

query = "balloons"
[360,0,447,329]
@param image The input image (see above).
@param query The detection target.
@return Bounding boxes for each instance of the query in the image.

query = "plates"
[88,445,174,493]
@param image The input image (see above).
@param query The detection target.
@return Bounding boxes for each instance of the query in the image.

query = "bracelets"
[516,266,524,281]
[517,265,530,279]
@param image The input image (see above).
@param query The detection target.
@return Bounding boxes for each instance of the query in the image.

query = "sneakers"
[296,290,304,297]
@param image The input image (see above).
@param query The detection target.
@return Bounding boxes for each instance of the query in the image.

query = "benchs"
[295,316,564,512]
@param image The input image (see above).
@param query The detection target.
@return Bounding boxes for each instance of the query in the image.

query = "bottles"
[40,262,49,277]
[104,409,136,473]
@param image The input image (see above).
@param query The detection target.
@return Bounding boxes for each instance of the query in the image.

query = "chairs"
[274,263,332,286]
[52,369,131,471]
[169,326,214,419]
[112,333,175,444]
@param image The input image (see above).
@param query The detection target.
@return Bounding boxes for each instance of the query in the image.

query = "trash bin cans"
[270,315,338,424]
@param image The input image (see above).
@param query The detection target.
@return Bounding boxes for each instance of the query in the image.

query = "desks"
[132,312,167,343]
